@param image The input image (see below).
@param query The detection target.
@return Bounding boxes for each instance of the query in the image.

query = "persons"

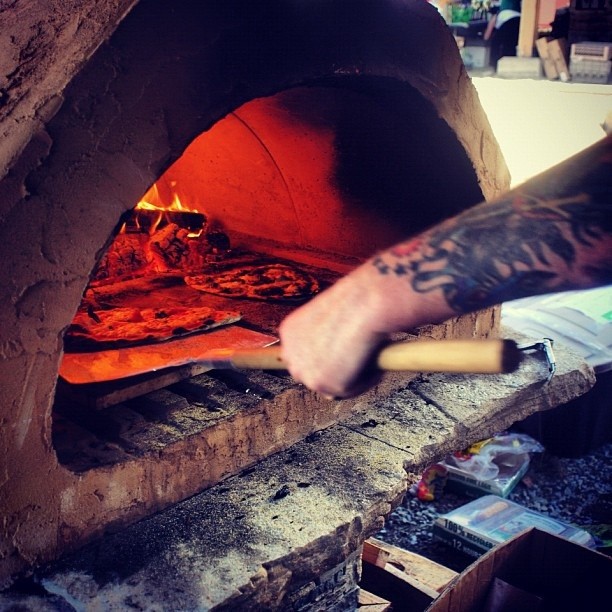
[278,133,611,400]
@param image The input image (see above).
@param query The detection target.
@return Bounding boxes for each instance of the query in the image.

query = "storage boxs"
[548,38,570,81]
[428,527,612,612]
[535,37,558,80]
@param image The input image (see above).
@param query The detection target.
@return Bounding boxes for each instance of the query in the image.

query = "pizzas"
[184,262,320,303]
[66,306,242,345]
